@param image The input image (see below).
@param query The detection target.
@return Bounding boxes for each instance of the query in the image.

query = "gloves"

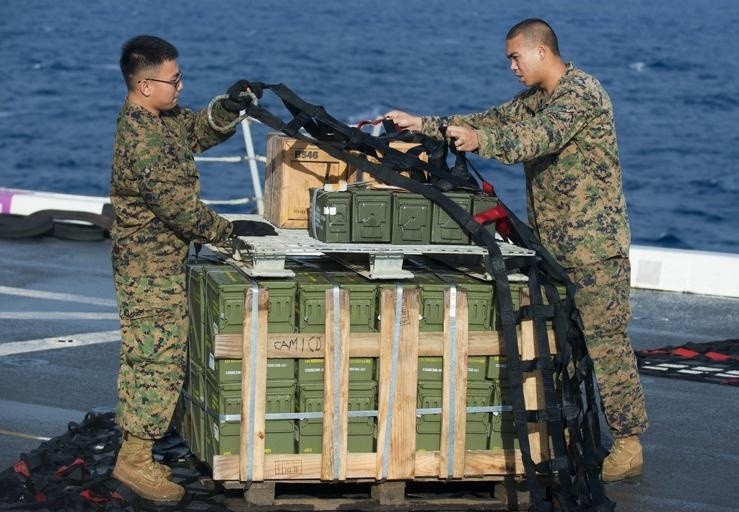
[229,219,277,240]
[218,79,263,110]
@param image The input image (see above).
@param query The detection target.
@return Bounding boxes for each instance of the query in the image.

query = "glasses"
[136,73,183,87]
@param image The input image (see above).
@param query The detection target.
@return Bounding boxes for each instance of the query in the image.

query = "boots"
[599,435,645,483]
[144,437,175,480]
[112,431,186,503]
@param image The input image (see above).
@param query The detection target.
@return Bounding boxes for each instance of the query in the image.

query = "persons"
[378,18,649,483]
[109,35,256,509]
